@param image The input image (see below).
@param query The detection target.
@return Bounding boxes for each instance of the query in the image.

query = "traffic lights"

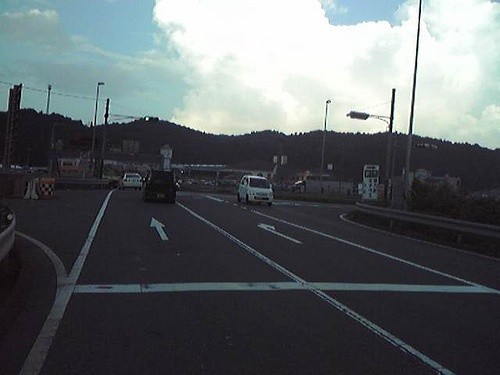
[143,116,160,124]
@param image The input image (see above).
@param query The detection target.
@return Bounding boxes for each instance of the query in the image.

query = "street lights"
[46,84,53,115]
[346,88,396,208]
[318,100,331,195]
[88,81,105,178]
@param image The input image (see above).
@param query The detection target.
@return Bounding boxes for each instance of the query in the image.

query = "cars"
[119,173,142,191]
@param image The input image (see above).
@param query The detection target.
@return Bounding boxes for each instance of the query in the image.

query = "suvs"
[237,174,274,206]
[140,169,177,204]
